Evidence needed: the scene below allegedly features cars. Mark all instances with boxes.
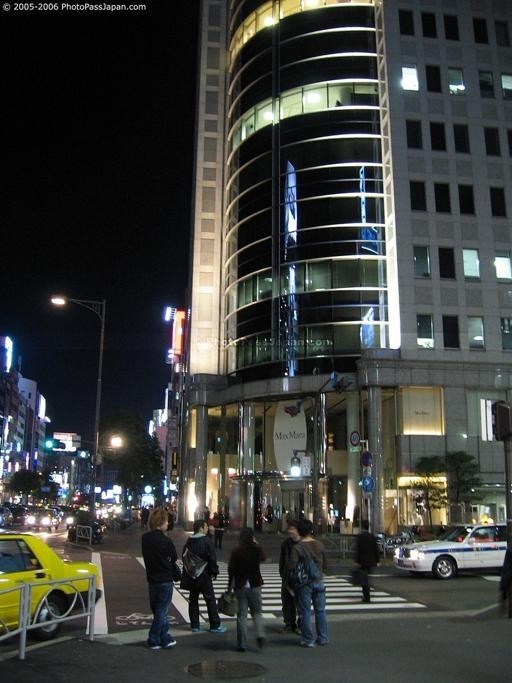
[0,534,102,635]
[393,522,508,578]
[0,502,124,532]
[370,531,406,553]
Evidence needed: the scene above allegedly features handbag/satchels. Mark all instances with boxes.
[207,517,220,527]
[146,546,322,617]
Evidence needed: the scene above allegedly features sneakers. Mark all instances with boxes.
[149,624,330,652]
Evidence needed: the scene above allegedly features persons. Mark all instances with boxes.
[214,511,225,548]
[142,510,180,650]
[228,527,266,652]
[132,505,174,532]
[256,504,287,536]
[182,519,227,633]
[436,526,467,541]
[194,507,201,522]
[288,519,329,647]
[202,507,211,538]
[413,526,425,536]
[311,520,321,537]
[278,520,303,635]
[355,520,378,603]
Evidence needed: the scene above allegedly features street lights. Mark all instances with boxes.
[291,449,319,535]
[51,295,106,540]
